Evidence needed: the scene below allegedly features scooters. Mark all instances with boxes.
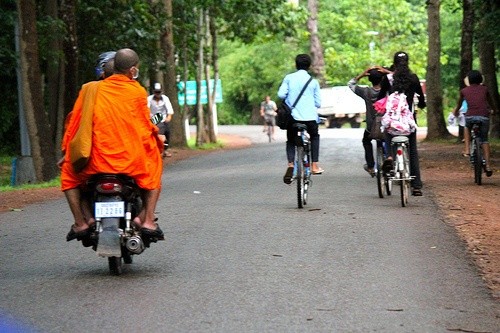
[88,113,170,277]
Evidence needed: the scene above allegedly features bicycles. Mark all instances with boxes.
[284,123,322,208]
[262,115,275,142]
[363,102,423,208]
[454,112,494,186]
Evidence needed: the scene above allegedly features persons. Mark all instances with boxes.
[452,71,496,176]
[148,84,174,158]
[56,47,164,242]
[351,48,427,197]
[276,53,324,184]
[261,96,277,140]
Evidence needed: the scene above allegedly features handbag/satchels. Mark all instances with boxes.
[70,115,94,171]
[274,102,294,130]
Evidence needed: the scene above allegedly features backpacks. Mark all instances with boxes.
[380,92,417,136]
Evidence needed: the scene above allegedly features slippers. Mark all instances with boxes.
[66,227,93,242]
[131,221,140,231]
[141,223,164,240]
[89,221,95,228]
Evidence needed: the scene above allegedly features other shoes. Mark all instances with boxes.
[486,170,493,177]
[459,153,471,159]
[364,164,376,177]
[411,188,423,196]
[283,166,294,184]
[312,167,324,175]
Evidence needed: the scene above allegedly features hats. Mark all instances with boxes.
[153,83,162,92]
